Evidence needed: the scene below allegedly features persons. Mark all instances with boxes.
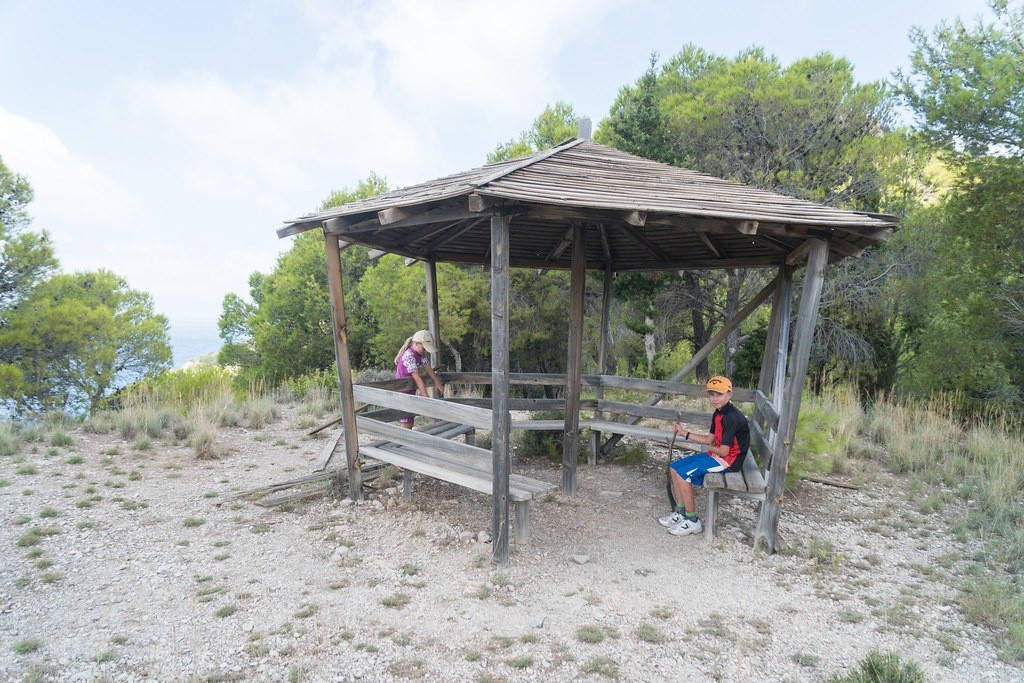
[659,376,751,536]
[394,330,445,430]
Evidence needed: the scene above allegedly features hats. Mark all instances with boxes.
[412,329,438,353]
[706,376,732,394]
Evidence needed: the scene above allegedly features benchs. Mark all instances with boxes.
[351,372,779,542]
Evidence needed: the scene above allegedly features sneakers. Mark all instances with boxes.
[668,517,702,536]
[658,512,685,528]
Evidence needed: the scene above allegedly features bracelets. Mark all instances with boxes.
[685,432,690,440]
